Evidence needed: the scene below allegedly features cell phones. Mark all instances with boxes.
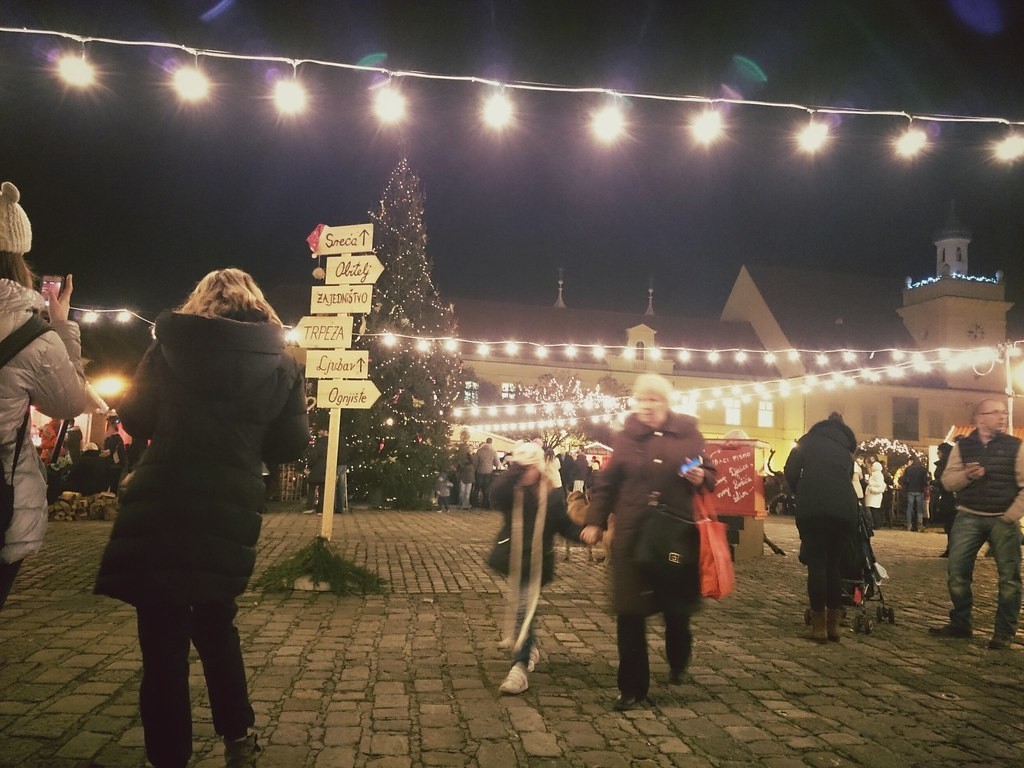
[679,455,704,476]
[37,273,66,320]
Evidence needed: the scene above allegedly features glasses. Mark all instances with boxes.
[982,409,1009,417]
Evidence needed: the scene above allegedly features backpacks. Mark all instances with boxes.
[0,311,56,550]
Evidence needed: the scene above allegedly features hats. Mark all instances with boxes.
[938,443,952,452]
[513,442,545,466]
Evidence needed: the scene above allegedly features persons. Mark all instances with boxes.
[0,182,85,622]
[765,398,1024,650]
[580,370,722,708]
[36,270,348,768]
[452,427,601,693]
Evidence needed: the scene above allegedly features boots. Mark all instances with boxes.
[826,607,840,641]
[812,607,828,643]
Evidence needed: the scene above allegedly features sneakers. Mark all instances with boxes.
[499,665,529,694]
[527,646,539,672]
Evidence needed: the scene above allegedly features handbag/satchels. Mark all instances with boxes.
[923,500,930,518]
[692,481,735,600]
[869,479,887,493]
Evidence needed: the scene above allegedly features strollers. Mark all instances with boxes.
[803,497,895,634]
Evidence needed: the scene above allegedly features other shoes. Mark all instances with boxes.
[989,635,1012,648]
[223,732,261,768]
[614,687,649,709]
[927,623,973,637]
[669,650,692,682]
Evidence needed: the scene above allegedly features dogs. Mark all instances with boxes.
[564,489,616,567]
